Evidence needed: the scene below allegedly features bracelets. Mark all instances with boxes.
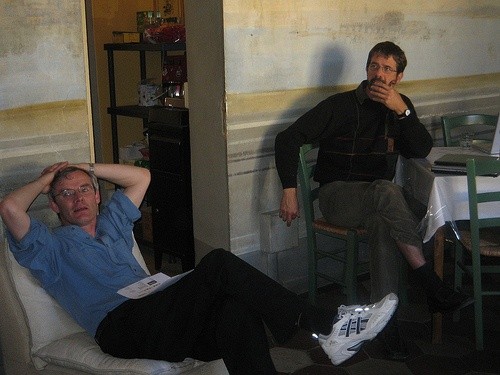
[88,163,95,174]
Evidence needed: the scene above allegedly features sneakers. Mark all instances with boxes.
[316,292,400,365]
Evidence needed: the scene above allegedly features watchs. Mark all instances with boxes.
[397,109,411,118]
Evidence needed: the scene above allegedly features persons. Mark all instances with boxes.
[275,40,477,360]
[0,161,399,375]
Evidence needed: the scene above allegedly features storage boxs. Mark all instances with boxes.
[111,32,141,43]
[164,97,185,107]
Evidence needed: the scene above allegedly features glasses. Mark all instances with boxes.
[54,185,97,201]
[369,62,397,75]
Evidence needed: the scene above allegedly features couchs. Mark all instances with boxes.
[0,203,228,375]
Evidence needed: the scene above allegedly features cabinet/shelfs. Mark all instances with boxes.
[102,42,193,272]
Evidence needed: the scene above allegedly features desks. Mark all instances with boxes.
[394,145,500,345]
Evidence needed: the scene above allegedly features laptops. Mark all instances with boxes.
[431,154,500,177]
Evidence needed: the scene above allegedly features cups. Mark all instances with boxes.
[459,132,474,150]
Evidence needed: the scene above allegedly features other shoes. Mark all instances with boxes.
[380,315,410,359]
[405,271,476,312]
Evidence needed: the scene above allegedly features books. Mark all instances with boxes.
[112,31,141,44]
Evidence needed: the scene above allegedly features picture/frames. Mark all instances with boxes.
[153,0,183,17]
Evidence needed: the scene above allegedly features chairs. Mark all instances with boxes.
[295,142,371,307]
[441,112,499,147]
[440,154,500,351]
